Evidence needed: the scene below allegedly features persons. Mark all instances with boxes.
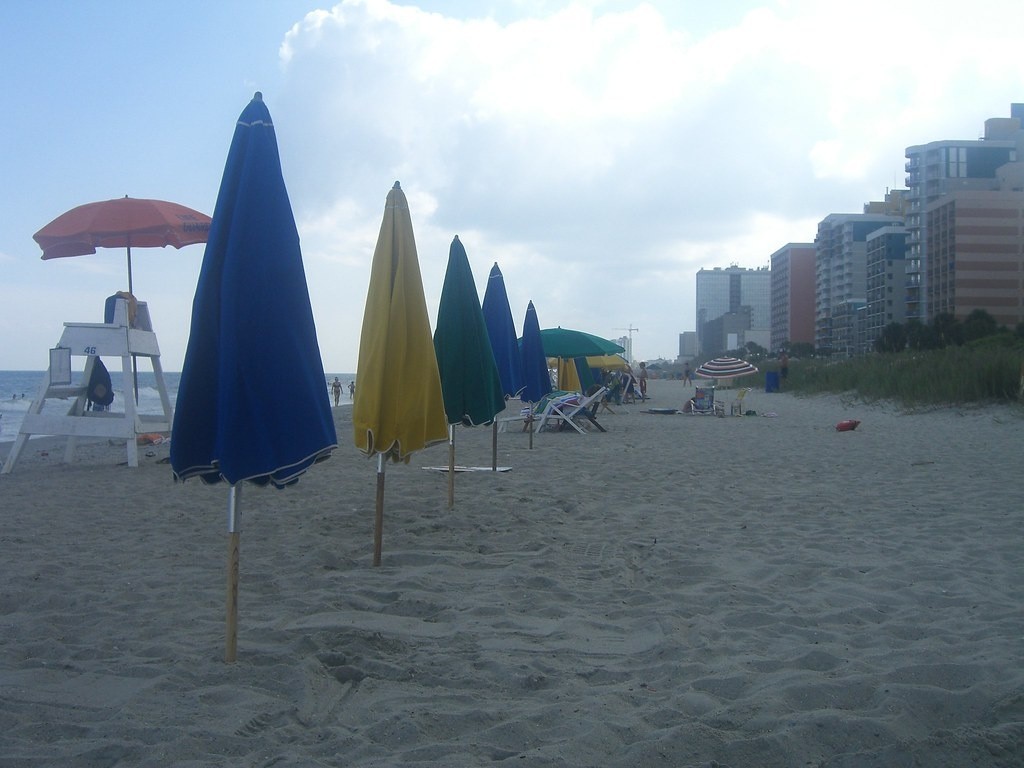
[778,348,789,393]
[639,362,648,402]
[619,372,636,404]
[331,377,343,407]
[348,381,355,399]
[682,397,705,413]
[605,375,622,406]
[683,363,692,387]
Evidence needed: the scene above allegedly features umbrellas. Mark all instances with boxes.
[695,355,759,402]
[482,262,552,471]
[353,181,450,567]
[433,235,506,506]
[170,92,338,662]
[32,194,212,407]
[517,326,638,424]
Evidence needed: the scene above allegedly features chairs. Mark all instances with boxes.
[498,371,638,435]
[691,386,716,416]
[104,294,125,324]
